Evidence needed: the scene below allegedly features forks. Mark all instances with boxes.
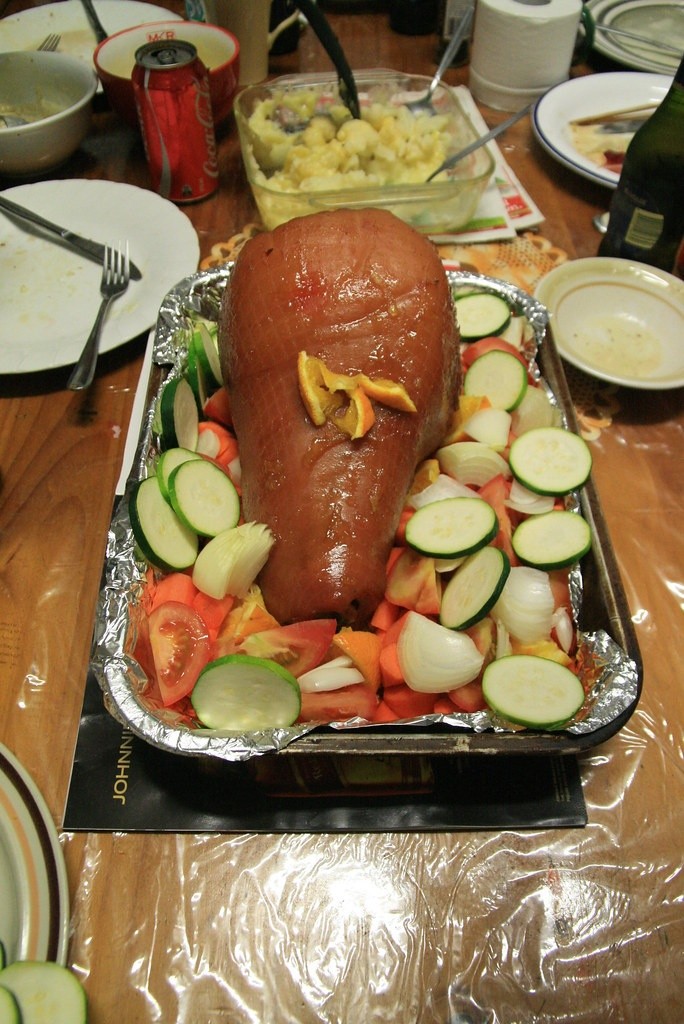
[65,238,131,392]
[36,34,61,52]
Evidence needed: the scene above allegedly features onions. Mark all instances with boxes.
[192,315,571,693]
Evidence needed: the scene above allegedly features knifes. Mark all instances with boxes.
[0,195,145,281]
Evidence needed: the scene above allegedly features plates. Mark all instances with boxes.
[578,0,684,75]
[91,273,647,765]
[0,0,185,94]
[534,255,684,390]
[528,72,675,187]
[0,179,202,375]
[0,739,71,973]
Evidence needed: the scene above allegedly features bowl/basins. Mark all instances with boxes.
[233,68,497,236]
[93,22,240,125]
[1,50,98,178]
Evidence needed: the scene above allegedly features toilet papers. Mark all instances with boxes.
[467,0,584,112]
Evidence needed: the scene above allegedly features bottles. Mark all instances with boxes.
[597,56,684,275]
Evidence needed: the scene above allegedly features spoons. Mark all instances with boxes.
[397,6,475,119]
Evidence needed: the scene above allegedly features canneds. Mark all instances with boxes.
[131,39,219,205]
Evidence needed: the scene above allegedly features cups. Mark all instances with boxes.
[185,0,271,85]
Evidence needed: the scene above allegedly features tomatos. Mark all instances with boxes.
[462,337,540,389]
[148,383,572,729]
